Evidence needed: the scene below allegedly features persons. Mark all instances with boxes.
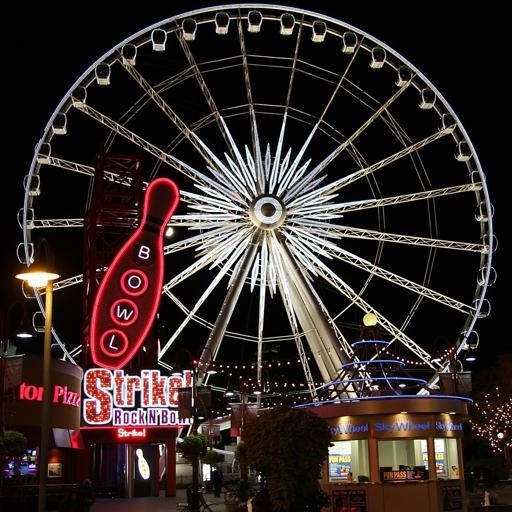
[212,466,224,498]
[79,474,96,512]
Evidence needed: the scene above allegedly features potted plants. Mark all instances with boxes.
[175,435,208,512]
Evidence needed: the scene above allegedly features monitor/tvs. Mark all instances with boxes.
[413,465,425,474]
[379,467,392,480]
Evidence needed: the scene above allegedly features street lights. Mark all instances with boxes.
[170,346,218,489]
[225,371,263,491]
[14,264,61,507]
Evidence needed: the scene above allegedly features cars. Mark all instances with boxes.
[465,461,500,490]
[0,460,38,475]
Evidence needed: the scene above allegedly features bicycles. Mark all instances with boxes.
[224,487,257,502]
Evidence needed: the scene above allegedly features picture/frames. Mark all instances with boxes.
[47,463,62,478]
